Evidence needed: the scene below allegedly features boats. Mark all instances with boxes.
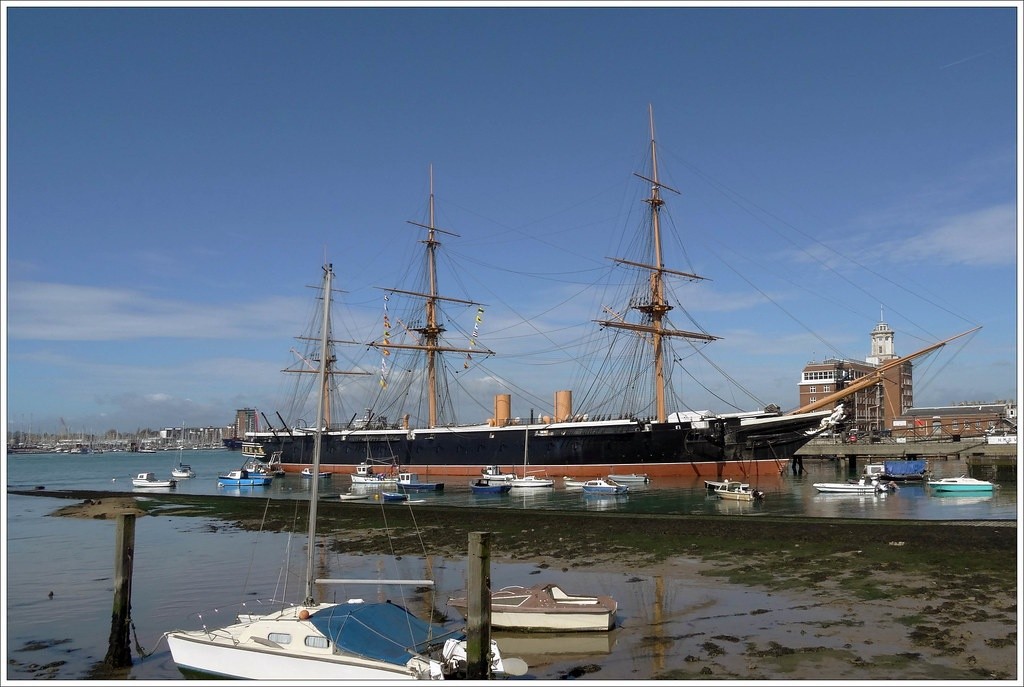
[446,585,617,631]
[132,455,993,502]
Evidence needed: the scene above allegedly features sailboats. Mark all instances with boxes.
[164,238,502,679]
[244,104,985,486]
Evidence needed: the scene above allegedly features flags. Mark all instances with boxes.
[464,307,484,370]
[379,294,392,389]
